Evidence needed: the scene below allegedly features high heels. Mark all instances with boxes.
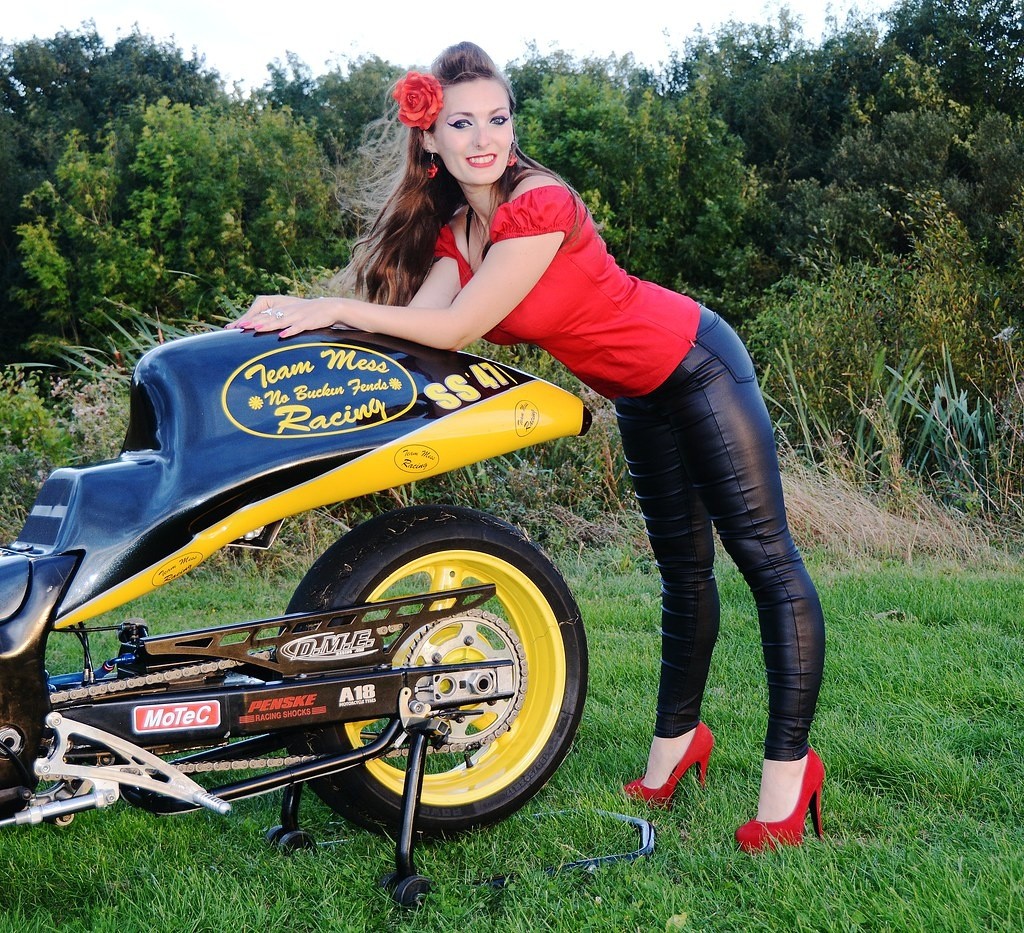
[623,720,714,811]
[736,745,826,856]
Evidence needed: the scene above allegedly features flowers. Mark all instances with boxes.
[393,71,444,131]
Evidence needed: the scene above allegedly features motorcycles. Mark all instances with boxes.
[0,325,661,911]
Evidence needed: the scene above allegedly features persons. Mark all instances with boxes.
[224,43,824,853]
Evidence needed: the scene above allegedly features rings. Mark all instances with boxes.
[275,311,284,319]
[260,308,272,315]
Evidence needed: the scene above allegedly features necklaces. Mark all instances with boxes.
[473,211,484,249]
